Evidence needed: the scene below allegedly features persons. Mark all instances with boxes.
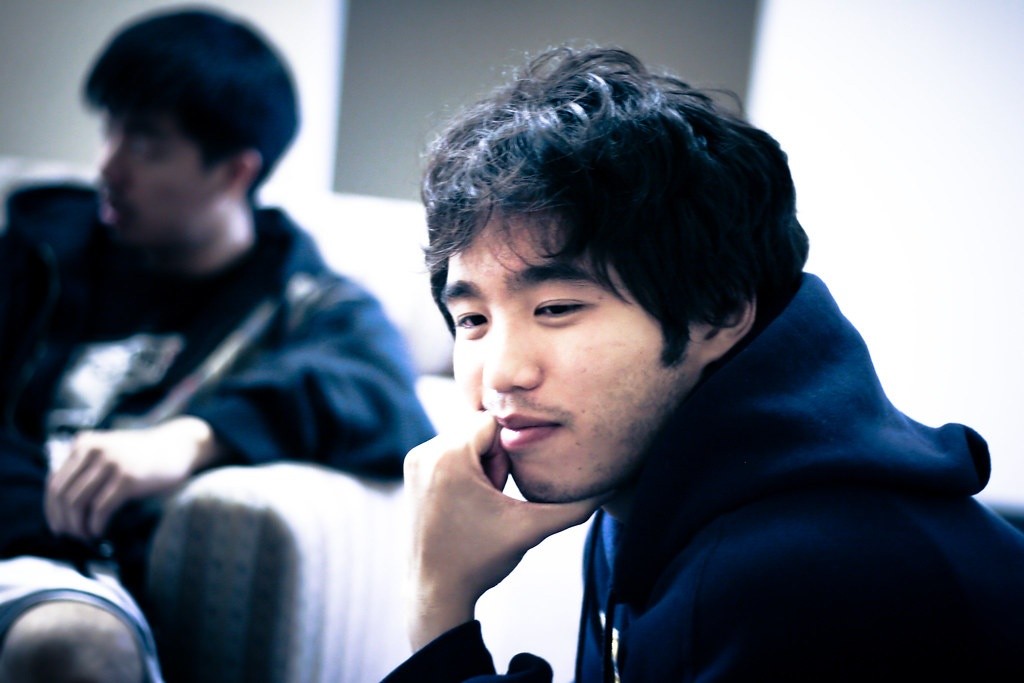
[375,43,1024,683]
[0,9,436,683]
